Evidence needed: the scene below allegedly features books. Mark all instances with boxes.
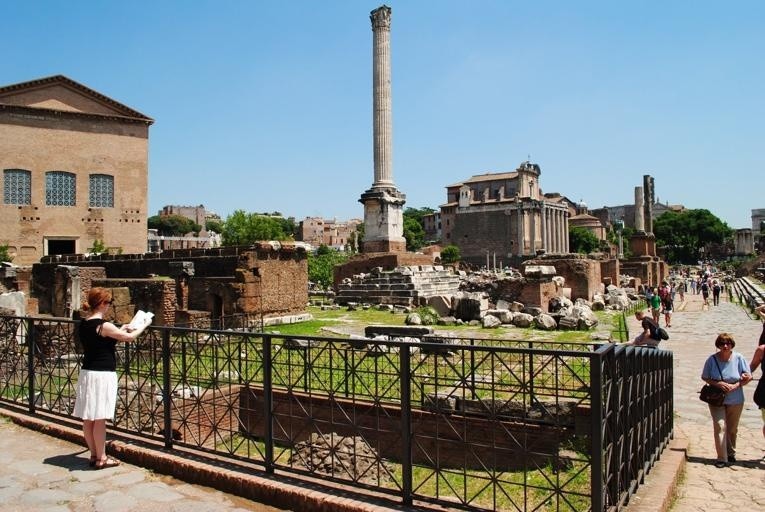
[125,309,155,331]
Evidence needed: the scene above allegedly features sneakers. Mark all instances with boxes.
[716,455,737,468]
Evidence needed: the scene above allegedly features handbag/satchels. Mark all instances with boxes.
[699,384,727,407]
[657,328,669,340]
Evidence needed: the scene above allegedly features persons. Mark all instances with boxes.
[645,264,727,328]
[71,287,153,469]
[702,334,753,469]
[606,309,663,352]
[739,327,765,435]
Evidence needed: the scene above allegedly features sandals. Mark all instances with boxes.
[89,455,121,470]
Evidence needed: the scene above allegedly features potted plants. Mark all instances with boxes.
[0,240,17,278]
